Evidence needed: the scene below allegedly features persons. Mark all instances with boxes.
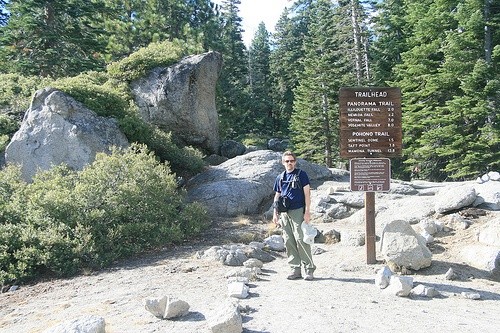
[273,151,316,280]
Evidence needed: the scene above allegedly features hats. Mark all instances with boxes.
[301,221,317,245]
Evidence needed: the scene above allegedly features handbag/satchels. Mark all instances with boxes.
[273,195,291,212]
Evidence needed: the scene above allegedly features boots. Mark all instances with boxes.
[286,267,302,280]
[304,269,314,280]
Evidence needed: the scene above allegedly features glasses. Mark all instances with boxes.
[284,160,295,163]
